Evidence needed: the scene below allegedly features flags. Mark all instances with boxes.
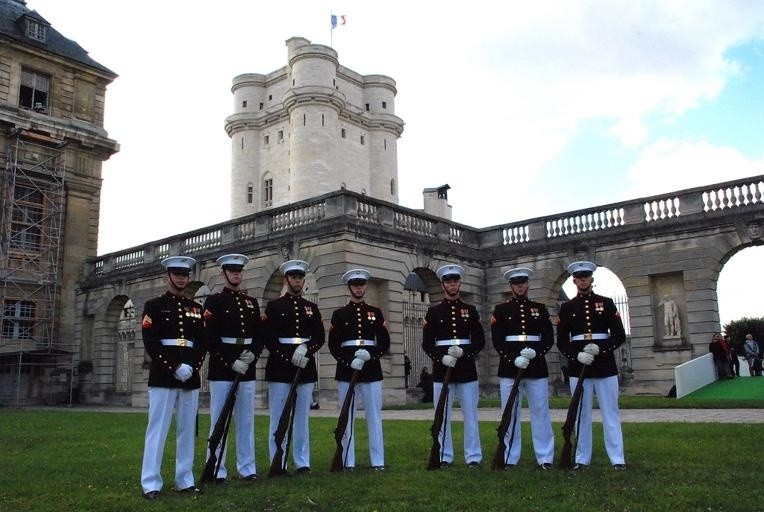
[331,15,346,28]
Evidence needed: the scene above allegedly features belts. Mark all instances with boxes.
[342,339,376,347]
[160,338,194,349]
[571,333,610,342]
[504,334,541,342]
[434,338,473,347]
[279,337,310,344]
[221,336,253,346]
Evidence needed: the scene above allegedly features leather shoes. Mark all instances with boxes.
[374,466,384,472]
[148,491,161,499]
[440,462,449,469]
[614,465,625,472]
[182,487,203,496]
[541,463,553,472]
[246,474,260,481]
[572,463,587,472]
[468,463,481,472]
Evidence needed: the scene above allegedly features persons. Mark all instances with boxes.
[709,333,740,379]
[405,350,412,389]
[328,269,389,471]
[422,264,486,470]
[556,261,627,472]
[490,268,555,472]
[203,254,264,483]
[743,334,763,376]
[657,294,677,336]
[141,256,207,500]
[419,367,433,403]
[261,260,325,474]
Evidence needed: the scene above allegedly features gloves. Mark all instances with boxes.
[520,347,536,359]
[172,363,193,383]
[354,348,371,361]
[350,358,364,371]
[583,344,600,356]
[240,349,256,364]
[448,346,464,358]
[576,351,595,366]
[290,345,310,369]
[442,355,458,368]
[514,357,530,369]
[231,360,249,375]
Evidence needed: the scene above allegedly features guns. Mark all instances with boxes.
[268,368,302,479]
[560,365,587,471]
[428,369,452,471]
[331,371,358,472]
[200,373,243,486]
[491,368,524,471]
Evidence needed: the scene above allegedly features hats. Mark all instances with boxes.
[568,261,597,277]
[436,265,466,283]
[504,268,534,284]
[216,253,249,271]
[160,256,196,272]
[341,269,371,285]
[279,260,310,276]
